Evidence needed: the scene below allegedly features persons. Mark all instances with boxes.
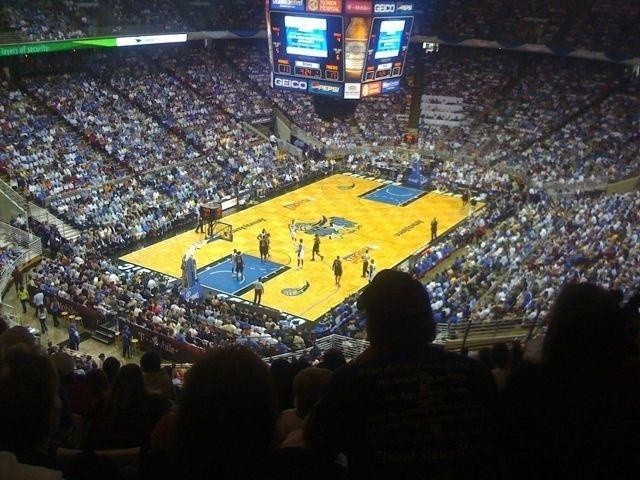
[1,1,640,477]
